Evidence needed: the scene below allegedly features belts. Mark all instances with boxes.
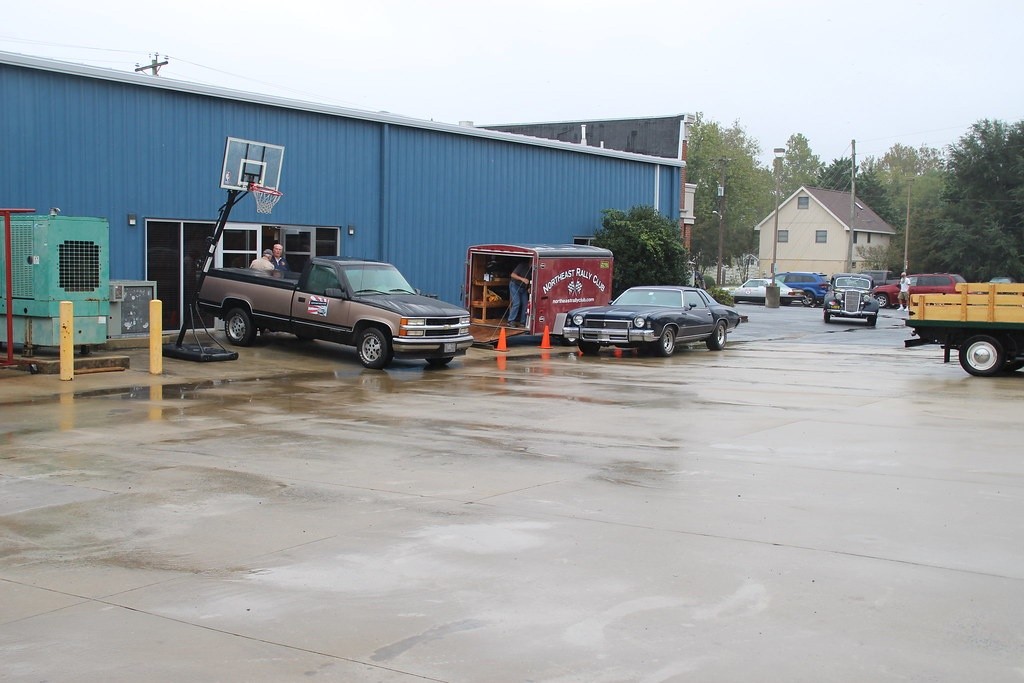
[512,281,525,288]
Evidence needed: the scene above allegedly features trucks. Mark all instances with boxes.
[904,283,1024,377]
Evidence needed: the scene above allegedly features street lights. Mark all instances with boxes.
[903,178,915,275]
[772,148,785,285]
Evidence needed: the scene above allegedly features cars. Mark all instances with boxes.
[988,275,1017,295]
[561,285,742,357]
[724,279,806,306]
[823,273,881,326]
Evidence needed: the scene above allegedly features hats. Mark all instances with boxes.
[263,249,273,256]
[901,272,906,276]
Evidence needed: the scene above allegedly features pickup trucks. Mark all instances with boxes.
[858,270,901,287]
[197,256,474,369]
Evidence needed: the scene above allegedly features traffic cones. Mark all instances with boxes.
[538,325,554,349]
[495,327,511,352]
[496,354,506,381]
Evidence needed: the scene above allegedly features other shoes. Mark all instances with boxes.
[897,307,903,310]
[520,323,525,327]
[904,308,909,311]
[507,321,516,328]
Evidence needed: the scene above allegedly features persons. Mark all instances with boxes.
[507,258,533,327]
[897,273,910,311]
[250,244,288,336]
[861,280,872,289]
[839,279,844,286]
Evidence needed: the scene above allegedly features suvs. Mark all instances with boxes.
[873,273,967,308]
[769,272,832,307]
